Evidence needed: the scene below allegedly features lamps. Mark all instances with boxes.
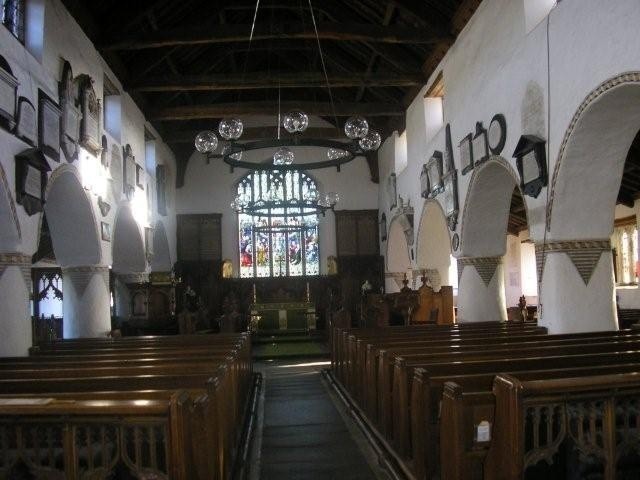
[231,190,339,216]
[195,1,381,172]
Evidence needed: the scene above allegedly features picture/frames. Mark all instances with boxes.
[460,134,472,174]
[451,233,459,252]
[39,89,62,162]
[101,222,110,241]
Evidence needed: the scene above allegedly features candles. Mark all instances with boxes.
[253,283,256,294]
[307,283,309,296]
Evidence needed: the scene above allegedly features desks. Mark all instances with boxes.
[262,302,305,329]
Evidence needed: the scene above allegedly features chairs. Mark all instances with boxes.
[331,309,640,480]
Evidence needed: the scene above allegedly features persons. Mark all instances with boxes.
[107,327,121,338]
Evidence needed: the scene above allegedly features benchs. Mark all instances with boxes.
[0,332,252,480]
